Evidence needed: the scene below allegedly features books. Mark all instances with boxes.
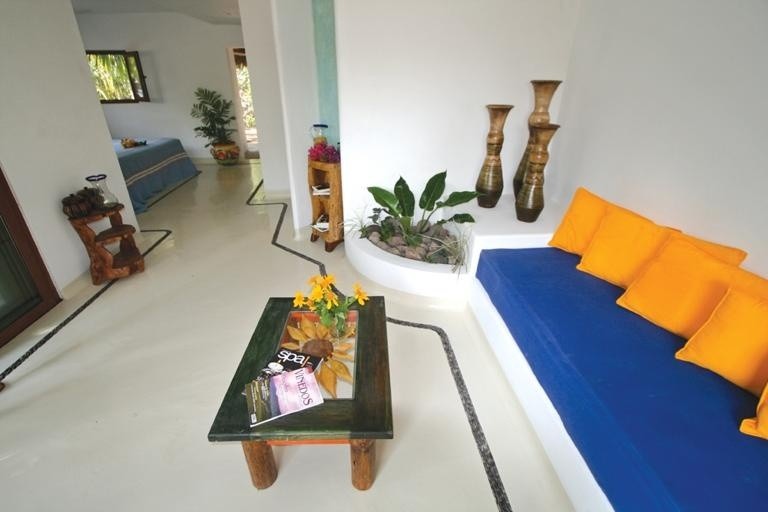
[244,366,325,429]
[241,345,324,399]
[309,182,330,235]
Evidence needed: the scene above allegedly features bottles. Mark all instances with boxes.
[84,173,119,211]
[308,123,328,148]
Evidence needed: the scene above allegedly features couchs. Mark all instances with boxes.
[470,243,768,512]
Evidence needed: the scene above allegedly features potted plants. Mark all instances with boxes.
[344,171,485,299]
[190,87,240,166]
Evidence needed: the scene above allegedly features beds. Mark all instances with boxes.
[113,138,198,215]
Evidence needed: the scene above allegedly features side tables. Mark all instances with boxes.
[445,185,564,312]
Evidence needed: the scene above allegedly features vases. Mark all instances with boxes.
[329,318,345,339]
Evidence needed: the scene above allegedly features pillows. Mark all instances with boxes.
[676,286,767,390]
[549,188,612,257]
[576,203,684,290]
[740,389,768,439]
[616,234,767,340]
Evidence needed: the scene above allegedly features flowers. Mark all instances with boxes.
[307,144,340,163]
[292,273,370,330]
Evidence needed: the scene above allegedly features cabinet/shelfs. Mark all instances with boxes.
[68,202,145,285]
[308,155,344,251]
